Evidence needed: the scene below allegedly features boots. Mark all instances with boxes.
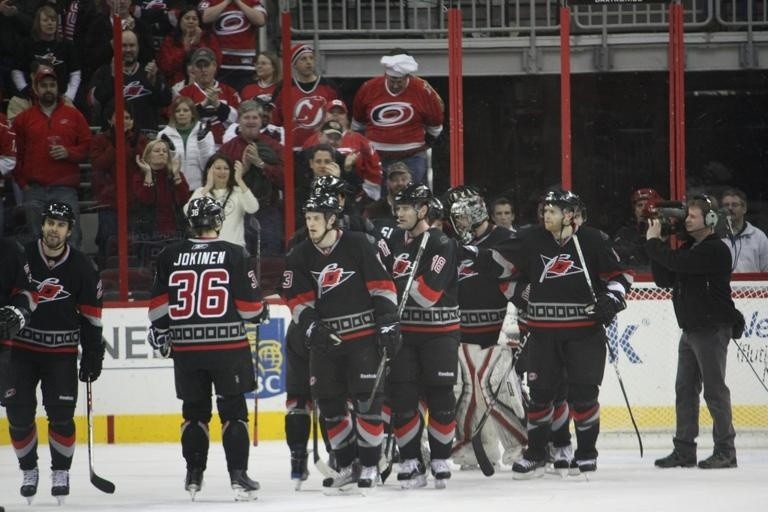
[699,451,737,468]
[655,437,697,467]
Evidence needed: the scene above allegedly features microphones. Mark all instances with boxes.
[687,223,714,232]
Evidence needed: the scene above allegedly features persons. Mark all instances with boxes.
[442,183,636,484]
[708,187,768,276]
[0,3,466,505]
[645,194,739,470]
[612,187,672,270]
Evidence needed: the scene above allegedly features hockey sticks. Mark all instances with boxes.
[86,376,117,493]
[312,409,332,476]
[249,215,262,446]
[572,233,644,465]
[472,330,532,475]
[356,232,431,414]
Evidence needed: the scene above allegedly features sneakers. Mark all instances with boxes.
[397,457,426,480]
[323,463,361,488]
[291,459,309,478]
[430,459,451,479]
[51,469,70,496]
[553,445,572,468]
[185,469,203,491]
[512,455,546,472]
[575,457,597,472]
[358,466,377,487]
[20,465,38,496]
[229,470,259,491]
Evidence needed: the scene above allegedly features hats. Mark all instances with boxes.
[255,93,276,110]
[322,120,343,136]
[324,98,348,113]
[387,161,408,176]
[291,44,312,66]
[191,46,216,65]
[35,68,58,82]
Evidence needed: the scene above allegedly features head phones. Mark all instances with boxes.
[694,193,718,229]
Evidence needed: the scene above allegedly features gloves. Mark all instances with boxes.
[303,319,345,351]
[79,343,105,382]
[0,303,32,340]
[594,290,626,327]
[377,321,404,364]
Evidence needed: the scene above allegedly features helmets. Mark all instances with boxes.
[41,200,76,227]
[302,193,339,212]
[444,184,481,216]
[631,188,661,202]
[573,205,589,226]
[543,190,580,207]
[311,175,345,193]
[449,195,489,232]
[392,181,432,204]
[184,196,225,229]
[428,196,444,211]
